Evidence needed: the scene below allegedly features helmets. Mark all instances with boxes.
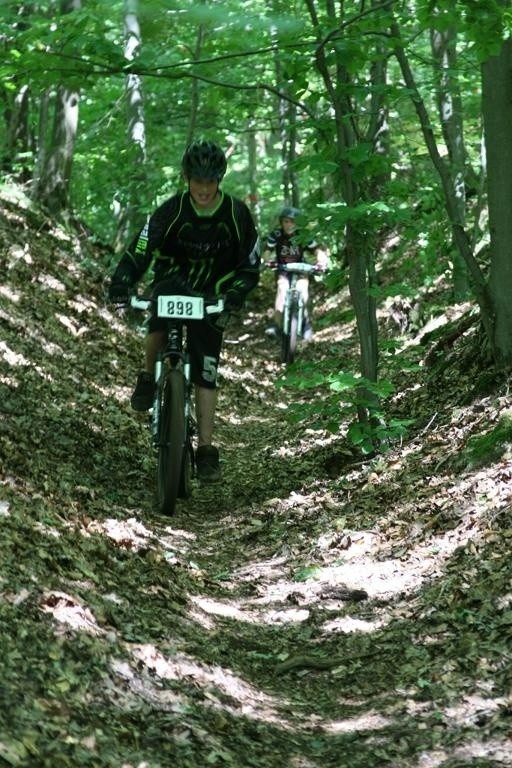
[180,138,227,180]
[280,207,300,220]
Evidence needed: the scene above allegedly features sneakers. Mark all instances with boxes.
[303,322,314,338]
[264,323,280,337]
[194,444,221,481]
[131,372,155,410]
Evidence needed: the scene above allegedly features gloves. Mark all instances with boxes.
[223,288,243,312]
[313,263,327,273]
[109,280,129,303]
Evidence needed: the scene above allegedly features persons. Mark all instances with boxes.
[265,206,330,343]
[108,139,265,486]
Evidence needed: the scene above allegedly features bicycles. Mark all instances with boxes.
[97,276,249,515]
[265,261,327,361]
